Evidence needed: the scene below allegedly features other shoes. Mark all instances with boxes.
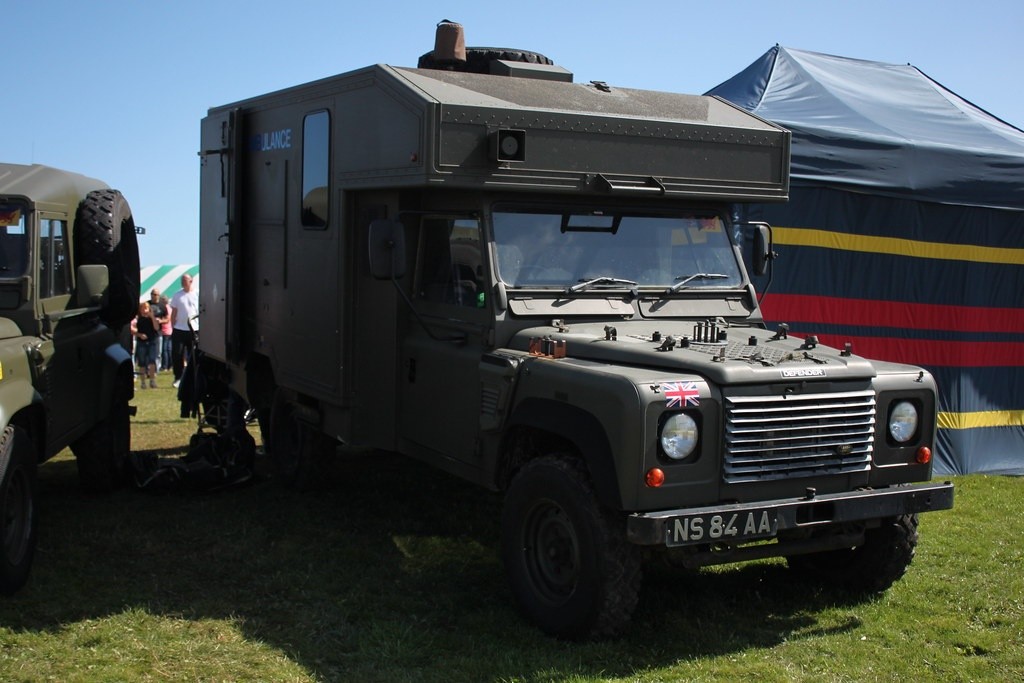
[141,384,146,389]
[150,381,157,387]
[172,379,181,388]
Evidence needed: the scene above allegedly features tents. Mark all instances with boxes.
[140,264,199,302]
[701,41,1024,478]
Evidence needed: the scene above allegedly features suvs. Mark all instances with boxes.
[0,162,146,598]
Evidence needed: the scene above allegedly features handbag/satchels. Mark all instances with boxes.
[189,425,223,452]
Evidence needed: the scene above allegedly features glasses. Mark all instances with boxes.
[151,294,158,296]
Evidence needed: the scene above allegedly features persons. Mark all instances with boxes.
[146,290,173,378]
[170,273,199,388]
[131,302,159,389]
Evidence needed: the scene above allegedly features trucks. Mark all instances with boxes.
[197,20,955,640]
